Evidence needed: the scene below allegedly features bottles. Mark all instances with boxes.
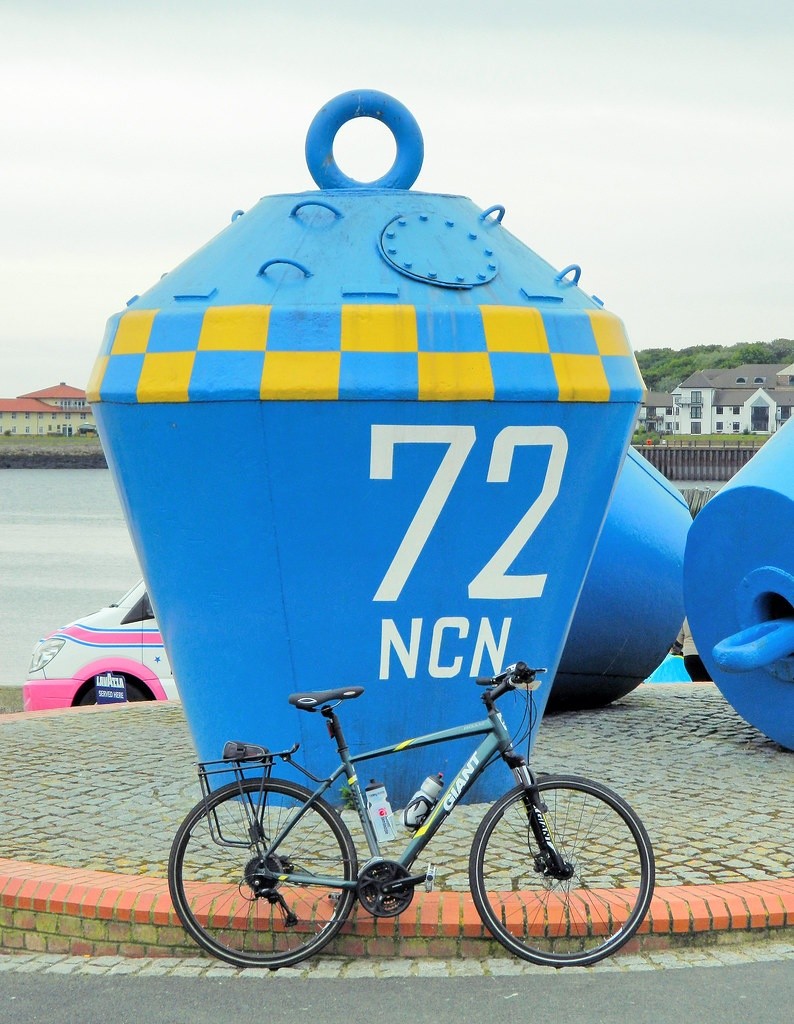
[398,772,445,833]
[365,778,399,844]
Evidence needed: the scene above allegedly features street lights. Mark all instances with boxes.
[672,404,679,441]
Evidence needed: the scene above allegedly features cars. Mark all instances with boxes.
[21,575,181,711]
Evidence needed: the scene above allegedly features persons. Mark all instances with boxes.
[672,616,713,682]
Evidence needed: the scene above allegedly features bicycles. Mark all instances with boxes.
[167,657,655,971]
